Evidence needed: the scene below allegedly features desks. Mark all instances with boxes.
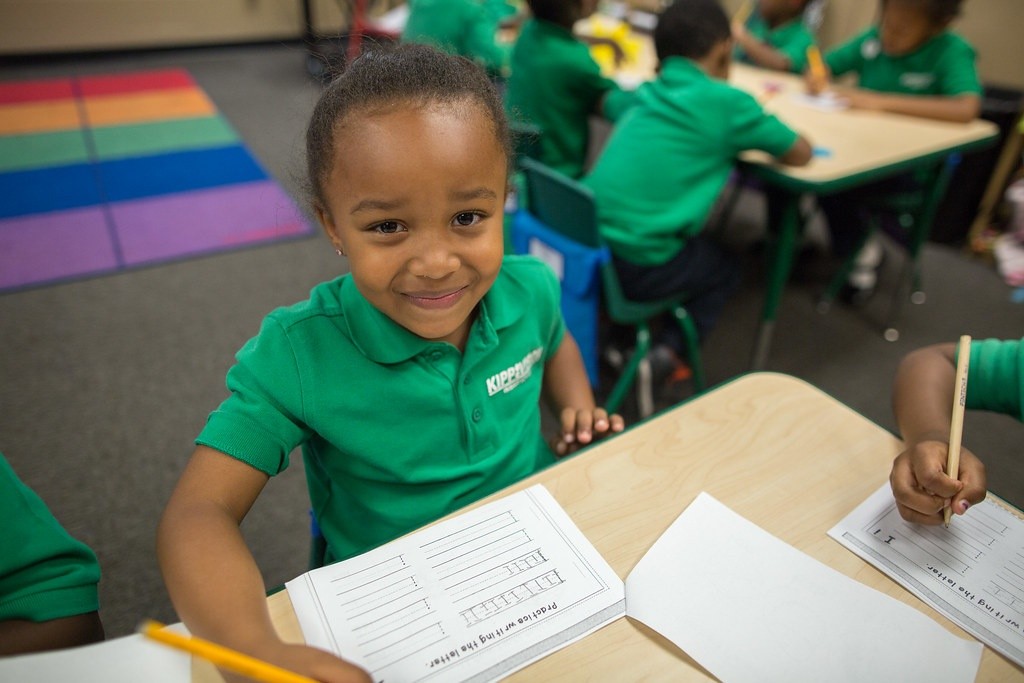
[192,371,1024,683]
[613,37,1003,372]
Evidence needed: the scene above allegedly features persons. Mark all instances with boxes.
[501,0,620,180]
[398,0,518,101]
[802,0,985,310]
[159,42,624,683]
[889,335,1024,524]
[728,0,819,278]
[581,0,813,389]
[0,451,102,658]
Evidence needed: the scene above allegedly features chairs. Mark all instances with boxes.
[506,114,715,425]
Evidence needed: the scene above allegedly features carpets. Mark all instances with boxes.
[0,68,319,296]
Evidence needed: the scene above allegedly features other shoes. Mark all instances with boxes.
[600,339,633,376]
[649,343,691,387]
[836,242,890,308]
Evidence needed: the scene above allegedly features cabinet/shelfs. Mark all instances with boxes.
[0,0,1024,90]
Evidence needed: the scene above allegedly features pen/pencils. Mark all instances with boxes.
[143,620,318,683]
[943,335,971,528]
[807,47,827,93]
